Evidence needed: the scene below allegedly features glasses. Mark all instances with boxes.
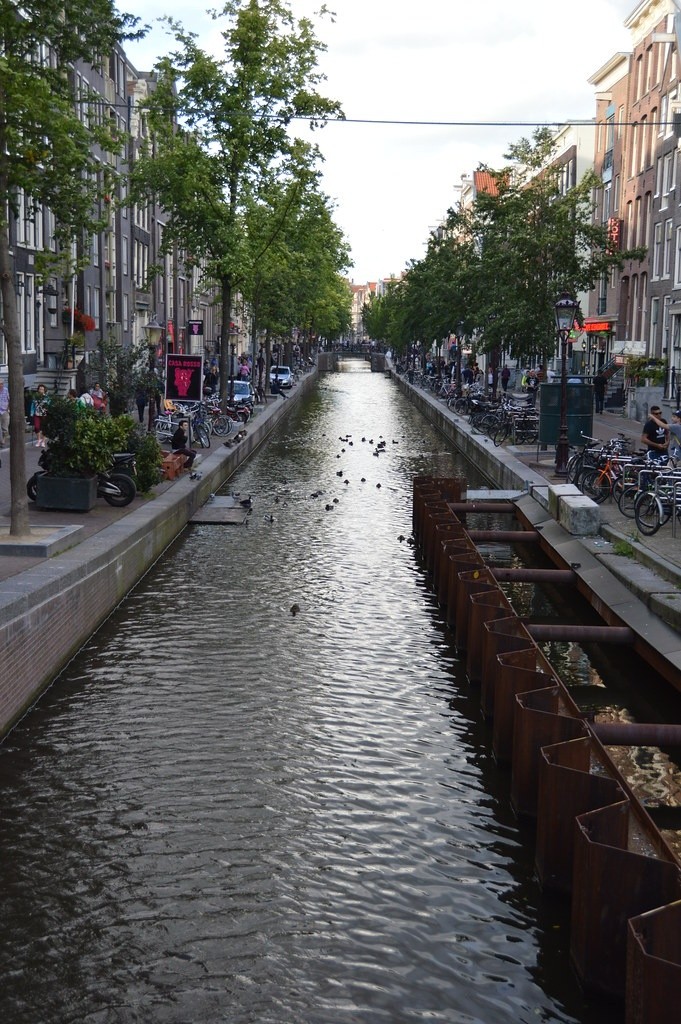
[651,412,662,415]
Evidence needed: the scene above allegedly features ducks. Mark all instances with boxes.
[290,604,300,616]
[189,430,448,547]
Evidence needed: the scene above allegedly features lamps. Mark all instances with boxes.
[582,338,587,354]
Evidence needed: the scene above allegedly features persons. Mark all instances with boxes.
[567,369,583,383]
[357,338,360,351]
[521,364,555,408]
[203,351,266,394]
[346,340,350,350]
[641,406,681,461]
[426,361,493,387]
[501,364,510,391]
[0,378,11,448]
[172,420,197,471]
[134,376,165,424]
[593,369,608,415]
[30,382,108,447]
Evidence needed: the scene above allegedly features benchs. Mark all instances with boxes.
[162,455,188,481]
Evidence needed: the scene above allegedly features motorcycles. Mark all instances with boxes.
[26,442,139,508]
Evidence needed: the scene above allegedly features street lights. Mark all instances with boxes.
[554,291,581,479]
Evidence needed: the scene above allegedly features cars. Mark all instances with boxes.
[154,395,254,449]
[270,366,295,388]
[227,380,256,402]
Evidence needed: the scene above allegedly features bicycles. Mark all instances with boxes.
[565,431,681,536]
[255,385,268,406]
[421,373,540,448]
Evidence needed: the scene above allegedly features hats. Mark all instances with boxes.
[672,410,681,418]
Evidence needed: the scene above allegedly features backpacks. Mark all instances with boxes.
[84,397,92,407]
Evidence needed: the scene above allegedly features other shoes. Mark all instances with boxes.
[184,466,197,472]
[1,441,6,448]
[596,410,603,414]
[35,442,46,448]
